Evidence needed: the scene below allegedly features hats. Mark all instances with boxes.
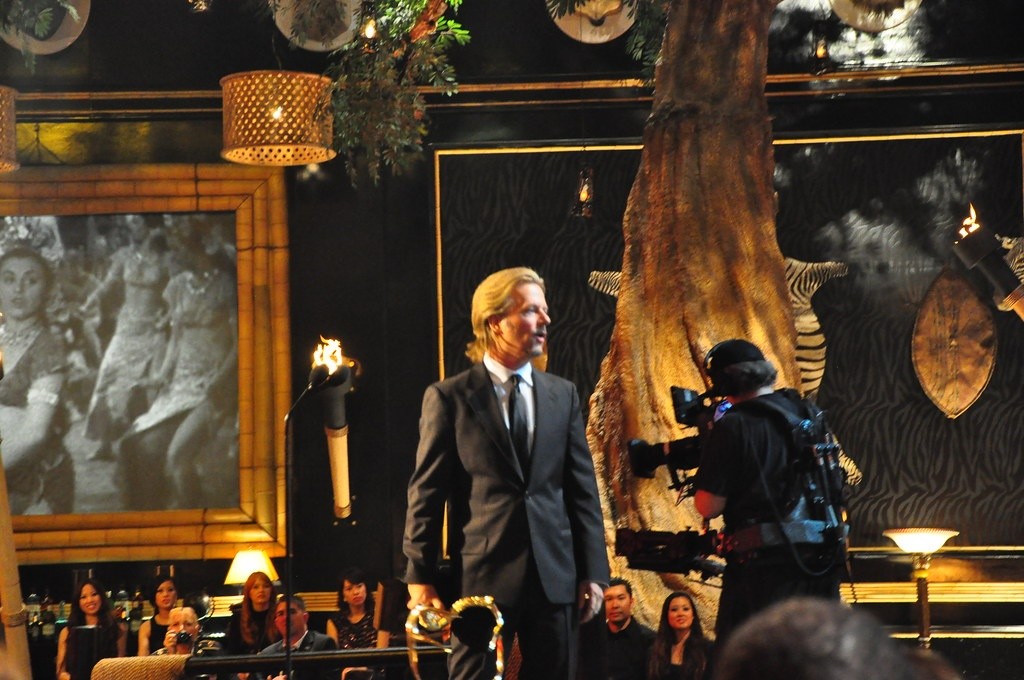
[711,339,766,370]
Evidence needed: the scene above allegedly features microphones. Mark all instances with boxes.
[306,363,330,388]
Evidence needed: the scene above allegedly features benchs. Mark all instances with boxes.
[840,573,1024,648]
[0,581,383,680]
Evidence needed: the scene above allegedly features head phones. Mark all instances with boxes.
[703,340,737,396]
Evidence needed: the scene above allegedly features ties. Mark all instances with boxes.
[509,374,529,470]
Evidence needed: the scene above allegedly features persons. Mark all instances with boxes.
[403,266,612,680]
[135,573,178,657]
[0,213,241,514]
[326,563,378,649]
[55,576,129,680]
[221,571,279,680]
[576,577,658,679]
[248,594,341,680]
[695,338,849,634]
[149,607,201,656]
[644,591,715,680]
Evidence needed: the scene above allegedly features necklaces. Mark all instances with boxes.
[0,321,46,347]
[186,268,220,295]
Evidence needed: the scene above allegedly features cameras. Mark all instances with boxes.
[174,631,191,643]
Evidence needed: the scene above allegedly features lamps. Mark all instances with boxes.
[881,527,960,652]
[216,0,339,168]
[224,552,281,595]
